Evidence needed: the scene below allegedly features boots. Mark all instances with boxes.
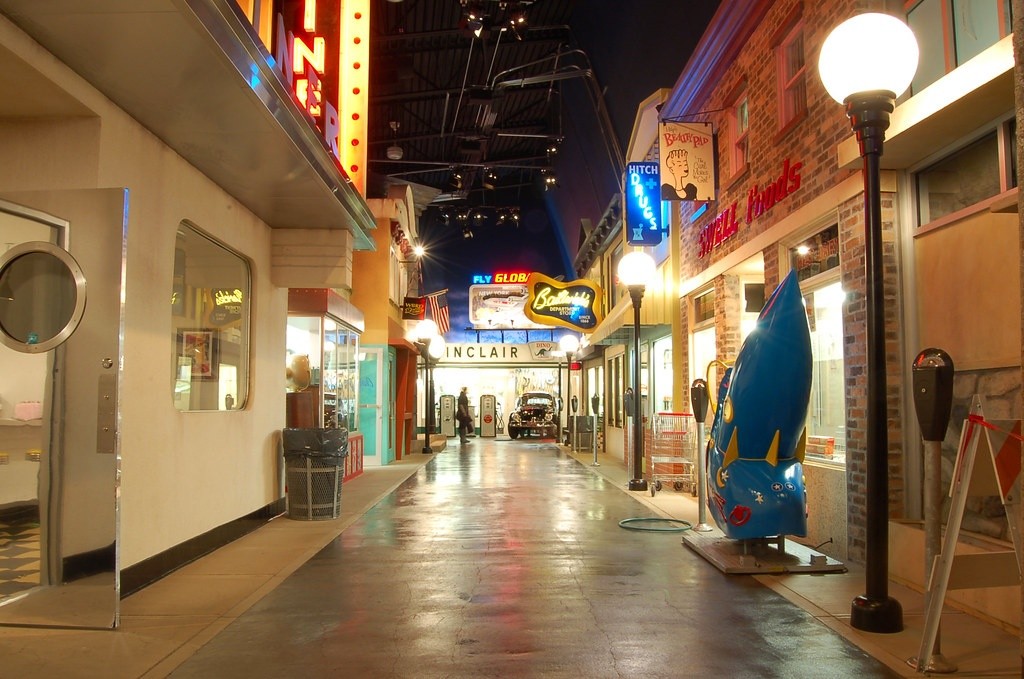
[460,433,470,443]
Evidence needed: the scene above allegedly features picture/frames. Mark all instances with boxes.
[175,328,220,382]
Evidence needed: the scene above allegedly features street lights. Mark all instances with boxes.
[816,11,921,634]
[416,317,439,455]
[558,335,580,446]
[427,334,446,433]
[617,250,657,491]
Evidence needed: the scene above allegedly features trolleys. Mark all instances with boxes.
[649,410,697,500]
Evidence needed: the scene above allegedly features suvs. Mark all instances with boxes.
[507,391,562,439]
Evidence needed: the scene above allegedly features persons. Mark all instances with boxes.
[456,386,474,445]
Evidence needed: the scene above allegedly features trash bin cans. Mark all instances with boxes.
[282,427,348,520]
[568,416,594,452]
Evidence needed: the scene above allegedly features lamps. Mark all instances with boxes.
[435,134,567,241]
[386,120,403,160]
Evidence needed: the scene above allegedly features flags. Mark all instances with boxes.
[428,291,451,335]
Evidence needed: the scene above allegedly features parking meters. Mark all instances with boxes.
[625,386,635,487]
[690,377,714,531]
[910,348,961,673]
[558,397,564,447]
[572,396,578,452]
[591,393,601,466]
